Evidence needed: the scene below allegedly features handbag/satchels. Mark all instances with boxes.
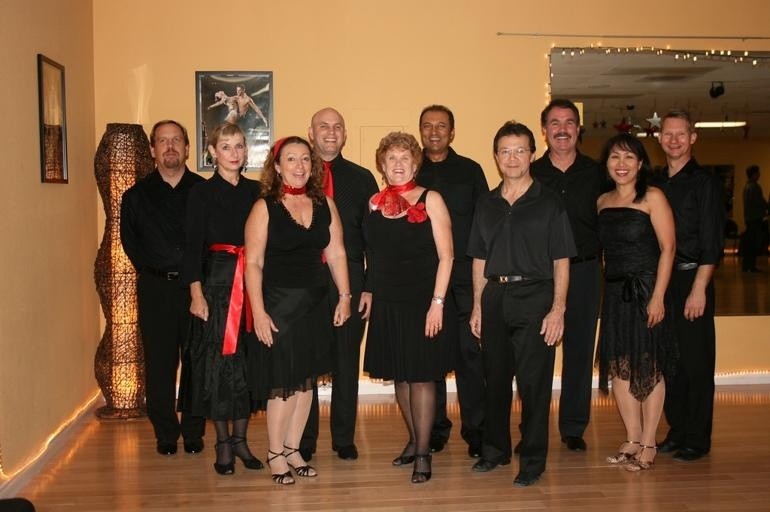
[430,294,447,303]
[338,290,351,301]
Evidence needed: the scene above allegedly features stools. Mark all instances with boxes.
[139,264,182,284]
[487,274,530,286]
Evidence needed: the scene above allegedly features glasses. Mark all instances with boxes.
[84,121,159,421]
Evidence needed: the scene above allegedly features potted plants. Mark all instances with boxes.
[194,70,274,173]
[38,53,68,184]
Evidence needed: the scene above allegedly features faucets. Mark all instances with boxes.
[607,441,658,472]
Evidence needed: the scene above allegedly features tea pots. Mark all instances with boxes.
[545,45,770,319]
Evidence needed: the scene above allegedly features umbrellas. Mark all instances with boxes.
[393,429,586,487]
[157,418,357,485]
[660,433,709,463]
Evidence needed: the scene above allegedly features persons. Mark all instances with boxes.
[516,99,595,455]
[358,131,457,484]
[649,110,727,462]
[468,119,575,488]
[119,120,208,455]
[206,90,241,129]
[410,102,489,456]
[223,85,271,125]
[242,135,354,484]
[183,120,266,476]
[300,107,380,462]
[741,159,767,275]
[595,136,678,481]
